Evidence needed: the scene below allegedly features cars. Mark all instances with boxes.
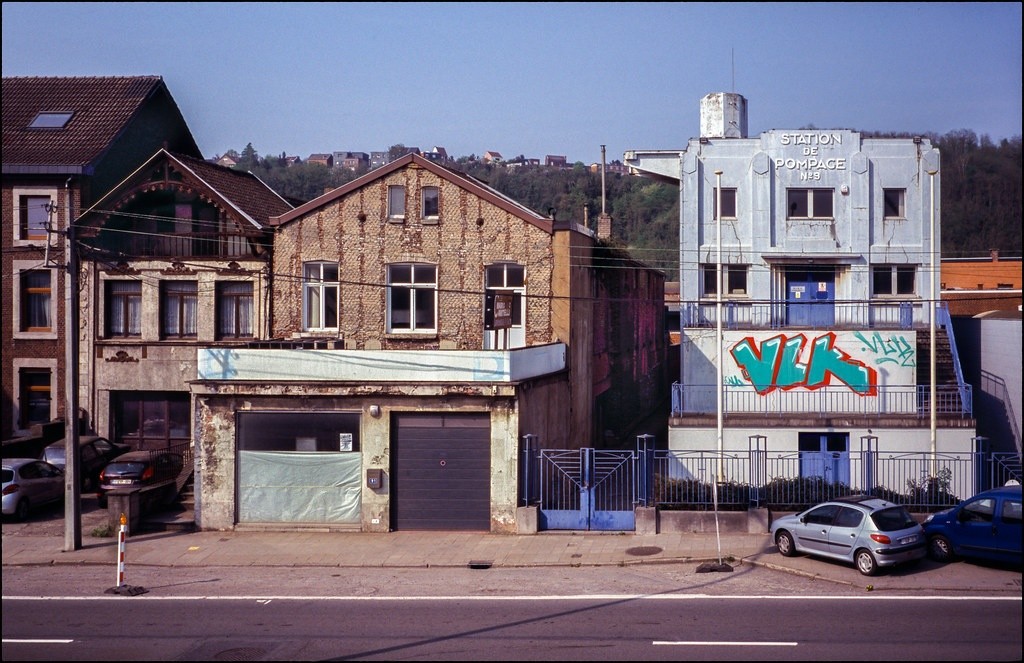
[0,458,66,520]
[39,435,131,493]
[97,451,182,509]
[921,485,1023,562]
[770,495,927,576]
